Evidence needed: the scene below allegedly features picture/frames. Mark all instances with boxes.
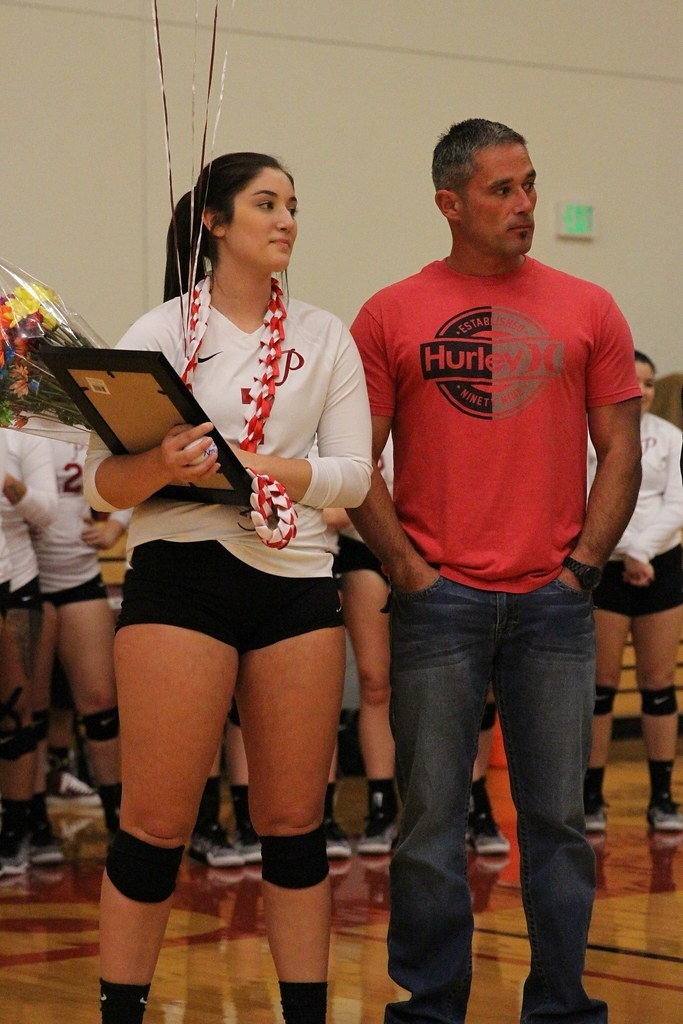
[41,346,253,504]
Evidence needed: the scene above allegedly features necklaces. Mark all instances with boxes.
[170,269,297,551]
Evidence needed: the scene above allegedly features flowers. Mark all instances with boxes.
[0,279,93,432]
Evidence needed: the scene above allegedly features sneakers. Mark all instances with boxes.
[465,809,510,856]
[329,862,350,886]
[46,767,102,810]
[327,817,353,859]
[1,829,29,874]
[189,824,246,867]
[646,800,682,830]
[587,833,605,846]
[2,878,26,888]
[209,867,262,884]
[362,857,390,884]
[233,827,262,864]
[475,857,509,874]
[583,794,607,831]
[649,834,678,854]
[33,828,66,865]
[35,869,65,886]
[358,814,405,853]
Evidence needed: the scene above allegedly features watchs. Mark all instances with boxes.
[564,557,602,590]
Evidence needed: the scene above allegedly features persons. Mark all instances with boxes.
[584,350,683,834]
[352,118,644,1024]
[0,406,133,876]
[191,425,511,866]
[81,152,373,1024]
